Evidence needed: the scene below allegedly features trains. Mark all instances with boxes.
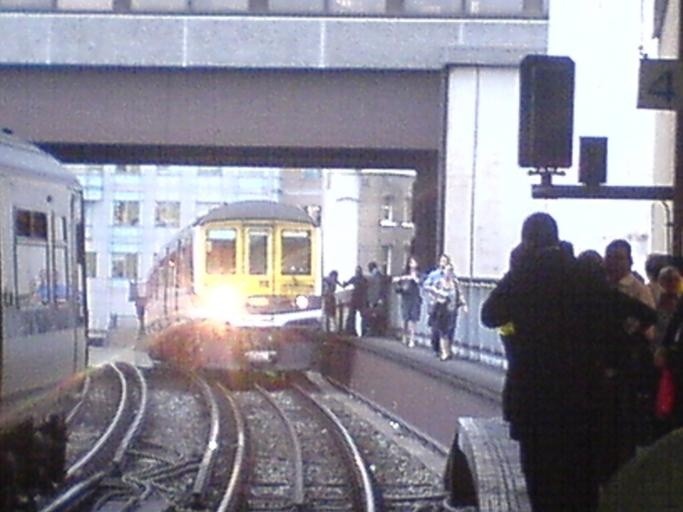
[142,199,323,380]
[0,128,89,511]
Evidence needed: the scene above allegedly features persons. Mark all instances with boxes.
[321,254,469,362]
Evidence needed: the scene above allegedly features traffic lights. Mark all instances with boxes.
[574,134,604,184]
